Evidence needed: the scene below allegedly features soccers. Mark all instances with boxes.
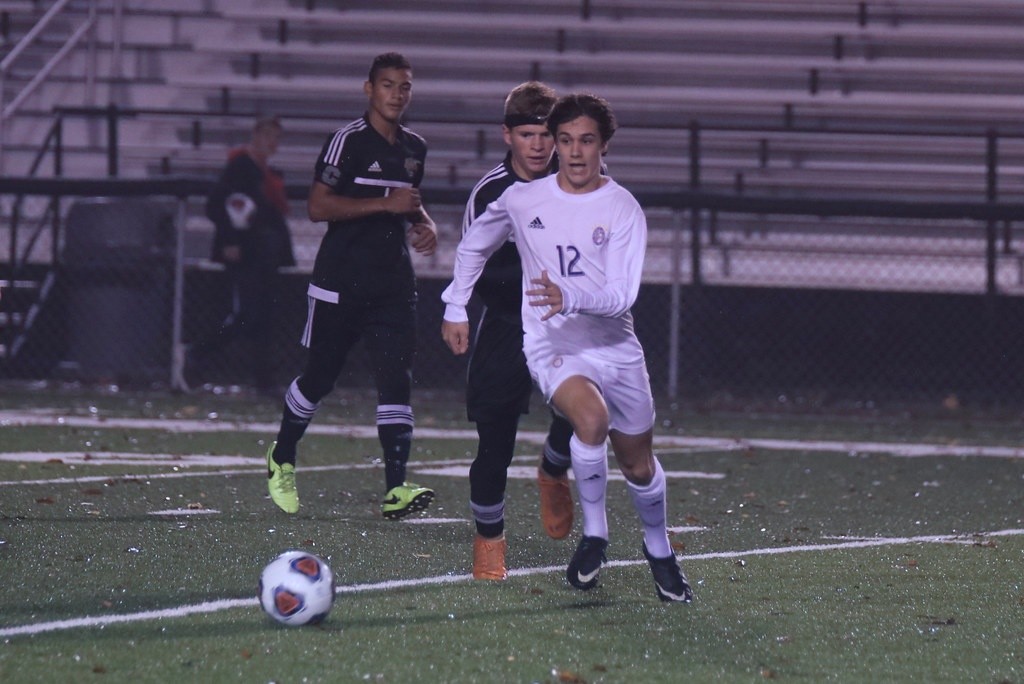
[256,549,336,627]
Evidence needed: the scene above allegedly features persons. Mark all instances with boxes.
[441,93,693,605]
[464,82,607,583]
[266,51,436,521]
[179,115,297,396]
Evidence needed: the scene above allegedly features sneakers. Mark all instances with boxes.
[267,440,299,514]
[382,483,435,520]
[566,533,609,590]
[471,532,508,582]
[536,452,572,537]
[642,536,693,605]
